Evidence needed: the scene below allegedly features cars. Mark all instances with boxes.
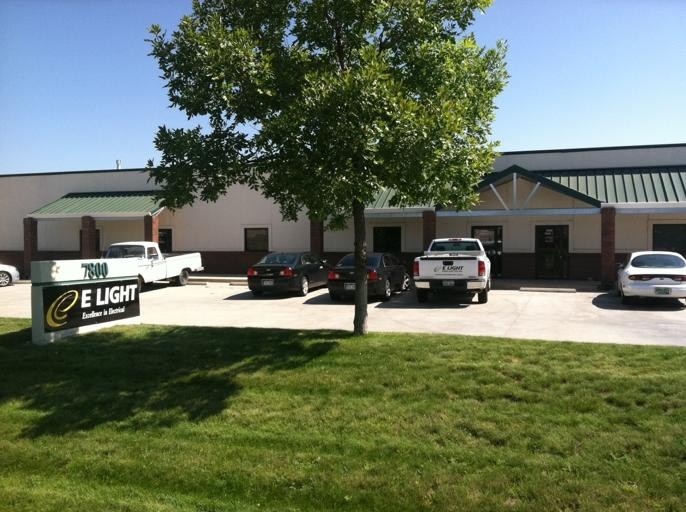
[0,262,20,287]
[246,250,329,298]
[615,249,686,304]
[327,254,413,300]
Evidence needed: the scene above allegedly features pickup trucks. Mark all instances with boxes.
[101,240,204,293]
[412,235,496,307]
